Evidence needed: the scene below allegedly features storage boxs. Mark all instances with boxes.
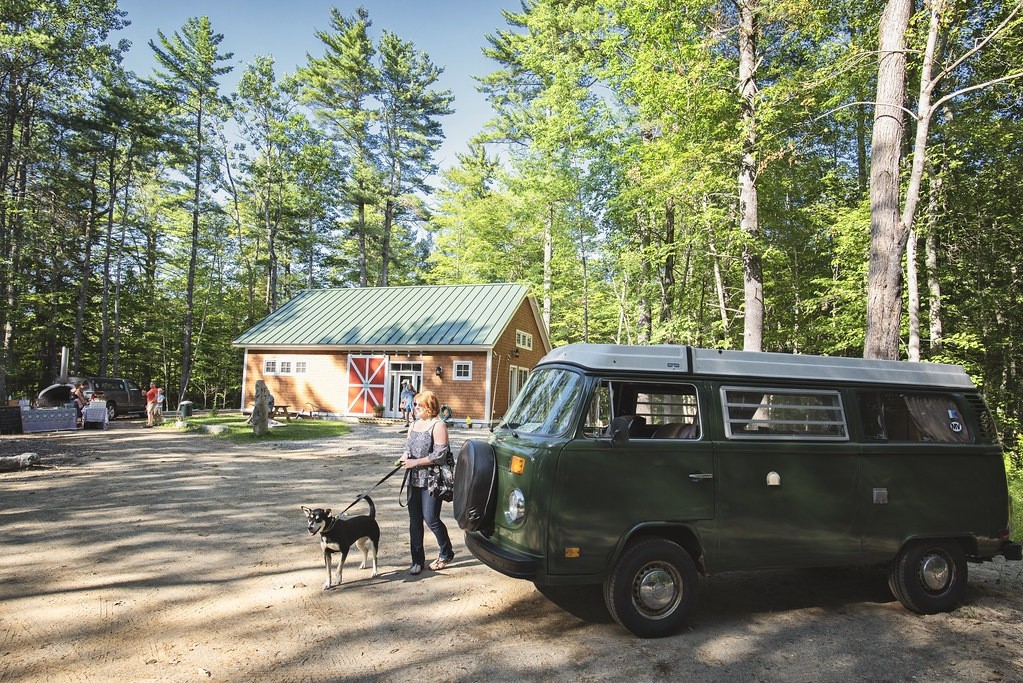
[9,399,30,411]
[89,401,107,408]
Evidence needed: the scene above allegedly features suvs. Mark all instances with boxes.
[52,376,148,422]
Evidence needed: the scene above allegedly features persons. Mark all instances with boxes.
[153,388,165,425]
[69,379,90,430]
[399,379,417,427]
[397,390,456,575]
[141,381,158,428]
[245,390,274,424]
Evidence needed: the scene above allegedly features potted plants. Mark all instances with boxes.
[372,405,385,418]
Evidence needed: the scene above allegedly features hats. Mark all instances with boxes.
[401,379,407,383]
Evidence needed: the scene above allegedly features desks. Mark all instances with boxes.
[271,406,292,423]
[21,409,78,433]
[82,408,109,431]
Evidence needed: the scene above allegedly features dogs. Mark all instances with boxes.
[300,493,381,591]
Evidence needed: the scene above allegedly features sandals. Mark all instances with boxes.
[428,551,454,571]
[409,562,424,575]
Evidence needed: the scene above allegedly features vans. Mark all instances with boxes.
[449,341,1022,638]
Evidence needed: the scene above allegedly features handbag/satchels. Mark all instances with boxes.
[427,421,456,502]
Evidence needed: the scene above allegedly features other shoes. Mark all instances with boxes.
[404,424,409,427]
[399,417,405,419]
[143,424,153,428]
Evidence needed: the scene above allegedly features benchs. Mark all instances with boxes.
[267,411,298,417]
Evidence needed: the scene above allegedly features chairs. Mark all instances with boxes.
[650,423,698,439]
[604,415,646,438]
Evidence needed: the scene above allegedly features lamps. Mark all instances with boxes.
[436,366,443,375]
[512,348,520,359]
